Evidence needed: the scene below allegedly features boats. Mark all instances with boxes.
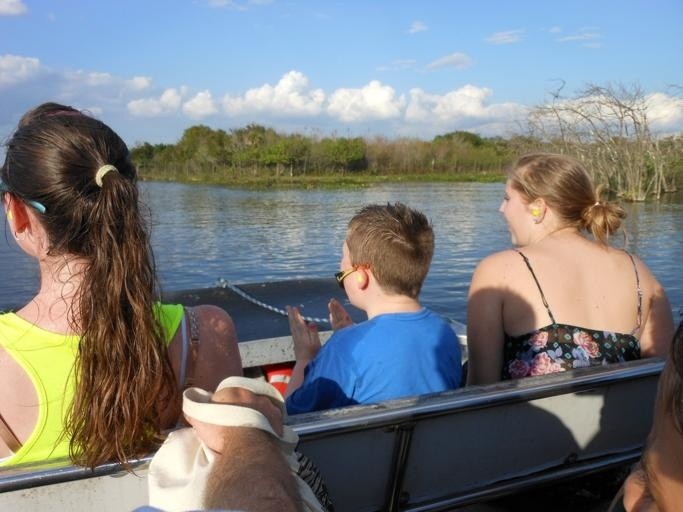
[0,273,668,510]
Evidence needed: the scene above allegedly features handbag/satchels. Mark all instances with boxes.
[147,375,335,512]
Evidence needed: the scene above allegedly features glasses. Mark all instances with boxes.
[335,267,357,288]
[1,170,49,214]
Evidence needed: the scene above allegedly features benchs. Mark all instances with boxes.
[1,356,666,512]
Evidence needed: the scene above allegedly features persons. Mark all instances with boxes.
[458,145,671,382]
[619,308,682,511]
[279,201,459,413]
[1,98,240,465]
[177,384,304,512]
